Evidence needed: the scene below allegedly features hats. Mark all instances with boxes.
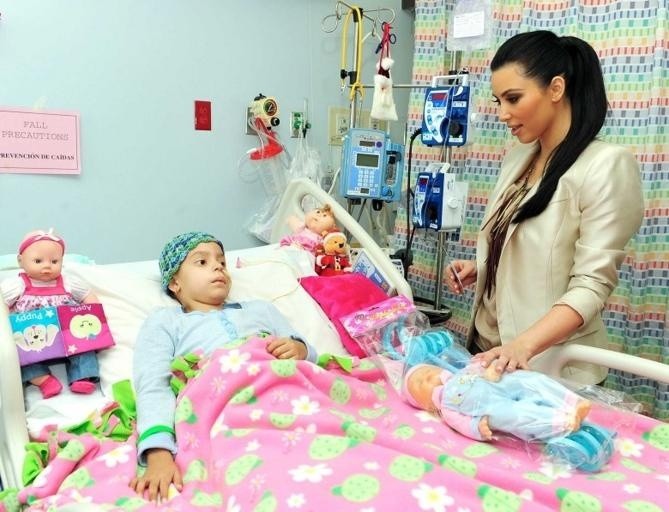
[157,230,223,295]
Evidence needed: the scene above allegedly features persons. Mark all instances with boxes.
[1,228,104,400]
[127,230,318,507]
[443,31,644,398]
[396,361,592,441]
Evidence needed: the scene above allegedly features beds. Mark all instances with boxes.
[0,174,668,512]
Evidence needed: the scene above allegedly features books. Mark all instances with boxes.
[354,250,397,298]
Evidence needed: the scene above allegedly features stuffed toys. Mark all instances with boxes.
[281,204,333,253]
[313,227,352,276]
